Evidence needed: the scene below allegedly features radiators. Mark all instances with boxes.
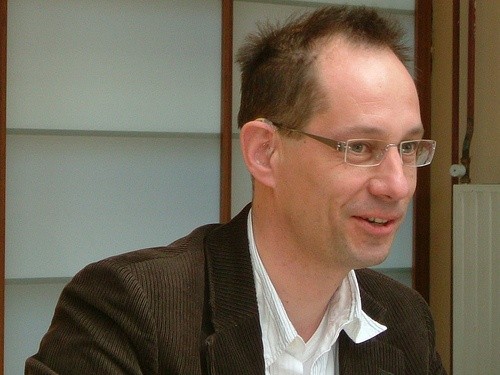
[451,182,500,375]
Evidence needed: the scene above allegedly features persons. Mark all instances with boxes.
[24,7,446,375]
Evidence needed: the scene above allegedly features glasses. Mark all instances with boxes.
[256,118,437,168]
